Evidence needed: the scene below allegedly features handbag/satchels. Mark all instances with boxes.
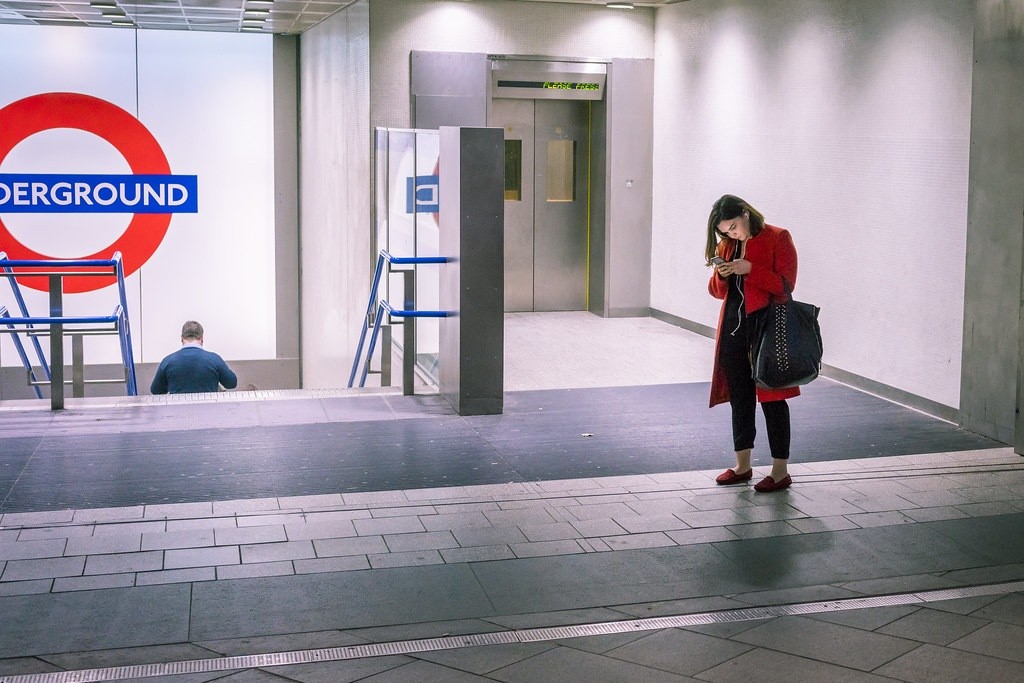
[747,276,822,390]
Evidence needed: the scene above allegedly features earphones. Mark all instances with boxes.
[744,213,749,219]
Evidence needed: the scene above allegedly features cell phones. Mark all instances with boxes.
[711,256,726,265]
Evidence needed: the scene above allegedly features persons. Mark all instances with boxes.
[704,194,798,492]
[149,320,238,395]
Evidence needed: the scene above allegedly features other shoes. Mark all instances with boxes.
[716,468,752,484]
[754,473,792,492]
